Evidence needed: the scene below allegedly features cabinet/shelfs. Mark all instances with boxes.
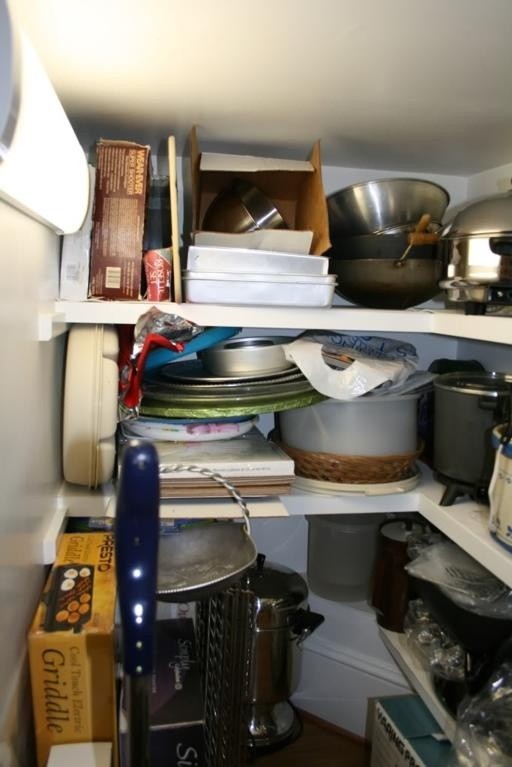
[39,297,511,767]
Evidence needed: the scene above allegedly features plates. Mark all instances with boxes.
[123,414,260,442]
[138,359,313,401]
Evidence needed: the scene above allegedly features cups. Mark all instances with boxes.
[377,516,428,633]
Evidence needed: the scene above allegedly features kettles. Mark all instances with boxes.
[193,551,326,749]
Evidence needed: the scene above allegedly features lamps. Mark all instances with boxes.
[0,0,91,238]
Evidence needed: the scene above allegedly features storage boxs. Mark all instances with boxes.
[183,125,335,258]
[26,531,124,767]
[84,136,154,303]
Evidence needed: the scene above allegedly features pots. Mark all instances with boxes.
[430,368,511,484]
[328,233,449,260]
[440,178,512,288]
[328,212,449,308]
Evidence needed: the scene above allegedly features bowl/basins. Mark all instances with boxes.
[197,335,297,377]
[326,177,451,232]
[277,390,424,454]
[202,178,290,234]
